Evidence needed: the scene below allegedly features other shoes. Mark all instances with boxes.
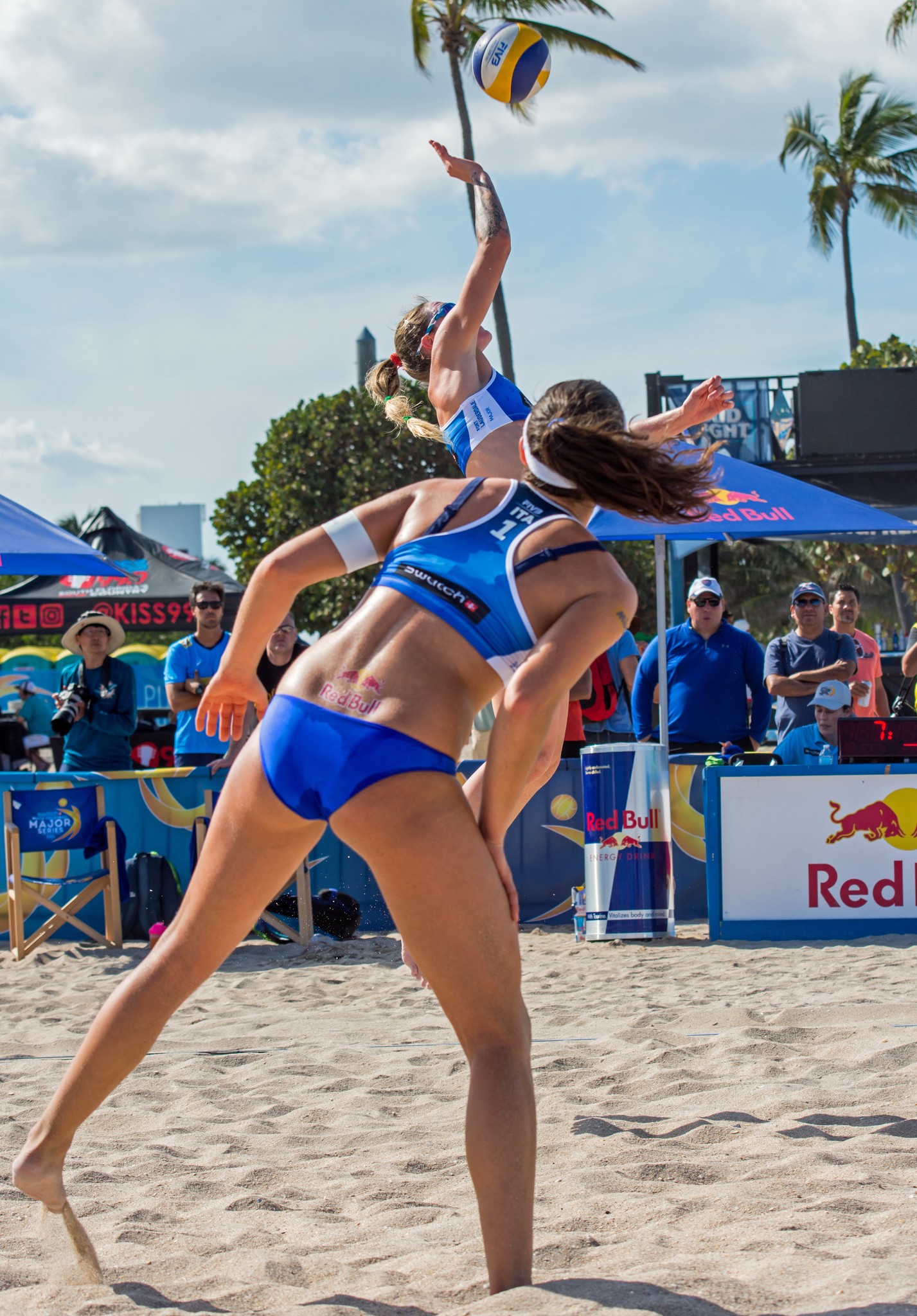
[36,763,51,772]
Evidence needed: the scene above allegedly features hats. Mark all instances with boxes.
[16,680,37,693]
[807,679,851,711]
[733,620,750,632]
[688,577,724,599]
[61,610,126,657]
[722,609,734,620]
[792,582,826,605]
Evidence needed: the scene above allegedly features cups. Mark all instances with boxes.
[149,922,167,950]
[857,680,872,707]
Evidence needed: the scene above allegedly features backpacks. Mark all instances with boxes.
[579,648,626,724]
[255,887,360,946]
[120,852,184,944]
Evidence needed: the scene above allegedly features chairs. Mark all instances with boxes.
[190,790,314,946]
[4,785,122,960]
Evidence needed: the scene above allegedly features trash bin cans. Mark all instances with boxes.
[579,742,675,941]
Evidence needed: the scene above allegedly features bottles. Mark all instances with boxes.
[819,745,833,765]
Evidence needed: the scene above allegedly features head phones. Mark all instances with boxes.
[22,681,29,696]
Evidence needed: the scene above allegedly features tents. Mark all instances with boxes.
[0,507,246,633]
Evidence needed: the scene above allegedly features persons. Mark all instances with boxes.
[11,379,730,1297]
[256,612,312,703]
[51,610,138,773]
[901,622,917,718]
[364,140,734,988]
[584,632,642,745]
[0,679,177,772]
[561,668,592,759]
[762,581,858,746]
[723,609,758,714]
[769,679,856,766]
[828,583,891,764]
[163,584,232,768]
[630,577,772,755]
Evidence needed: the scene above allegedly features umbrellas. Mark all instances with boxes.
[587,440,917,750]
[0,494,129,576]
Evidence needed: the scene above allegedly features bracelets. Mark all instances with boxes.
[322,514,381,576]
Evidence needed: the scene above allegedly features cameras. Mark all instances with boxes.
[51,682,92,736]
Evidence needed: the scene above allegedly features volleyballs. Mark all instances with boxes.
[472,22,551,104]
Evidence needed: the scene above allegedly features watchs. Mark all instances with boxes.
[194,684,205,699]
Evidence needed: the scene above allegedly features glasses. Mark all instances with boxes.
[194,600,223,610]
[274,624,296,633]
[794,598,825,608]
[853,638,865,659]
[690,596,721,607]
[416,302,457,357]
